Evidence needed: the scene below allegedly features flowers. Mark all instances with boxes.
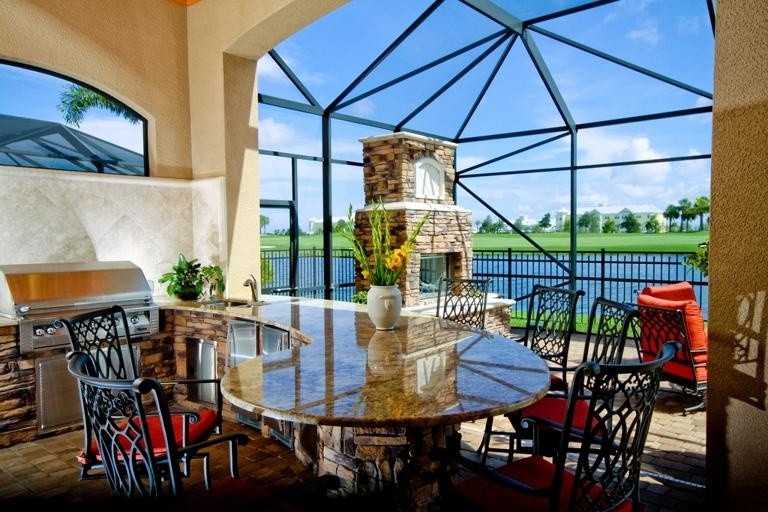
[332,194,432,286]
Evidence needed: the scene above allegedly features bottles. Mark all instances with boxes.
[367,330,404,377]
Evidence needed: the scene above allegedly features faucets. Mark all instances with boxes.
[242,273,259,302]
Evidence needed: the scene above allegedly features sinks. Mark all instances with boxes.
[214,298,271,309]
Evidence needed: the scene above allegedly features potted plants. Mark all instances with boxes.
[157,253,225,301]
